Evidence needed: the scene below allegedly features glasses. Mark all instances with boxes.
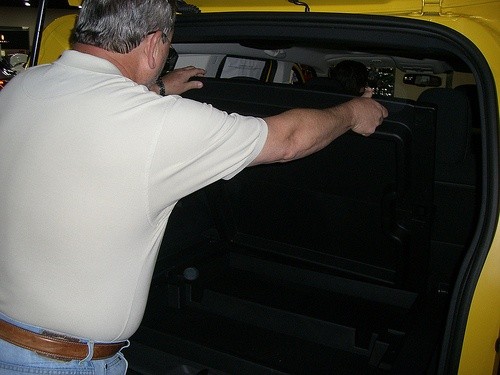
[145,28,177,61]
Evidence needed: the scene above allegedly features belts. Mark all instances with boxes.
[0,317,128,362]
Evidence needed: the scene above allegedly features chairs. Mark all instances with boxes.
[413,87,473,185]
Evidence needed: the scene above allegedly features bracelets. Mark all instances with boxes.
[156,78,165,97]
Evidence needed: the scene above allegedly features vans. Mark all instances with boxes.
[25,0,500,375]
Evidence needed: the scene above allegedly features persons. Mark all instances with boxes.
[0,0,388,375]
